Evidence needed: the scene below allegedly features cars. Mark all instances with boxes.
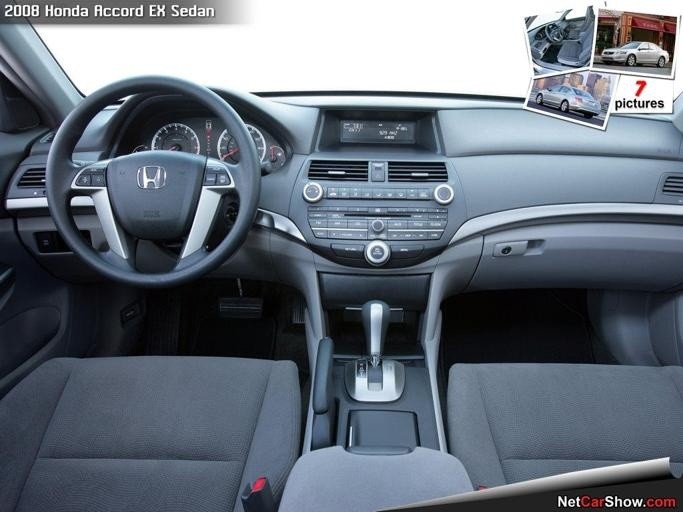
[600,41,669,68]
[534,85,601,119]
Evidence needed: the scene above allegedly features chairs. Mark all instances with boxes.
[1,356,682,512]
